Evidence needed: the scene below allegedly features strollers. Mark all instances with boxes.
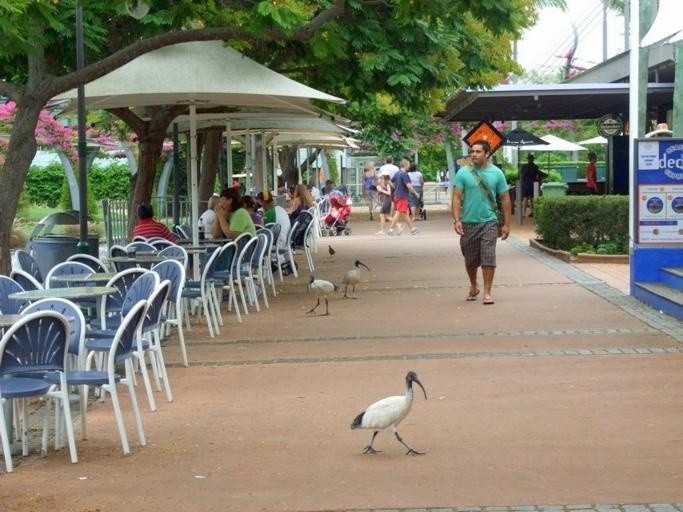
[408,189,426,220]
[319,183,352,237]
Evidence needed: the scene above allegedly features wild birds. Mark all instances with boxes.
[339,259,370,298]
[302,274,338,317]
[349,370,426,457]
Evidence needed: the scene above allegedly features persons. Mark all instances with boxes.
[586,152,601,196]
[521,154,539,217]
[361,156,425,236]
[452,140,511,306]
[132,203,177,247]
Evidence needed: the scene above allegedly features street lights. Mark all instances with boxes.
[71,1,154,261]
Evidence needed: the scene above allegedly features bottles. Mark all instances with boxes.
[197,217,205,242]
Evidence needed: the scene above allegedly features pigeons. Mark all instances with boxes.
[328,245,335,256]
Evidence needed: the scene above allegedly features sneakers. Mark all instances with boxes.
[246,286,262,302]
[375,223,421,236]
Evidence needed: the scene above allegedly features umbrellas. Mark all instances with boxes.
[501,125,550,146]
[578,136,614,145]
[518,134,589,174]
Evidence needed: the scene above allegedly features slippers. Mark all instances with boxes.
[466,288,479,301]
[482,297,494,304]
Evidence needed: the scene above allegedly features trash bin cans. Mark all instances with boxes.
[541,182,569,196]
[31,210,99,289]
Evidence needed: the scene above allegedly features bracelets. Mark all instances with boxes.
[454,220,459,224]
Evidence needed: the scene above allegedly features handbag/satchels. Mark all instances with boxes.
[495,209,503,237]
